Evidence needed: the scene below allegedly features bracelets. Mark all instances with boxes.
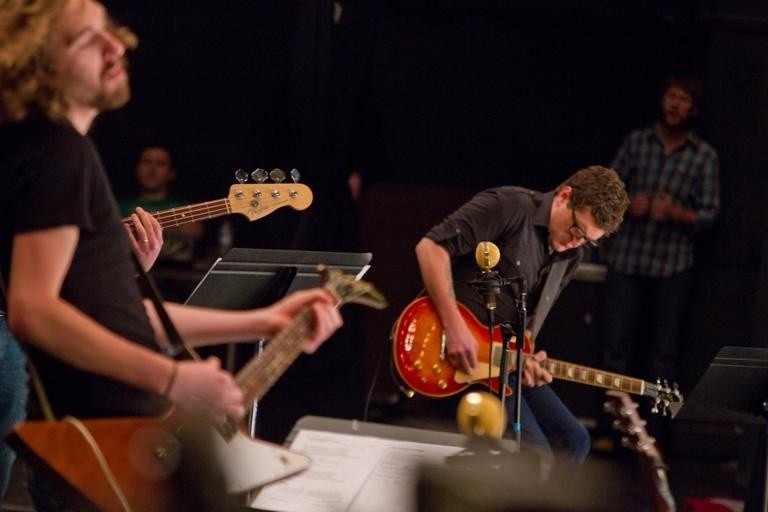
[163,360,178,400]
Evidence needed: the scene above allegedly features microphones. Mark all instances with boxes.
[456,390,509,439]
[474,241,501,270]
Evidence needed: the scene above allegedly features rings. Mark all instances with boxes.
[140,239,148,243]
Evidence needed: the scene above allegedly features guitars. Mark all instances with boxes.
[123,168,313,239]
[389,296,684,419]
[5,263,388,511]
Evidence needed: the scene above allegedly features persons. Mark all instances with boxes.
[588,78,720,457]
[118,139,203,307]
[1,1,344,512]
[415,165,631,481]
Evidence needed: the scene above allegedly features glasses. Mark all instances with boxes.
[568,206,604,252]
